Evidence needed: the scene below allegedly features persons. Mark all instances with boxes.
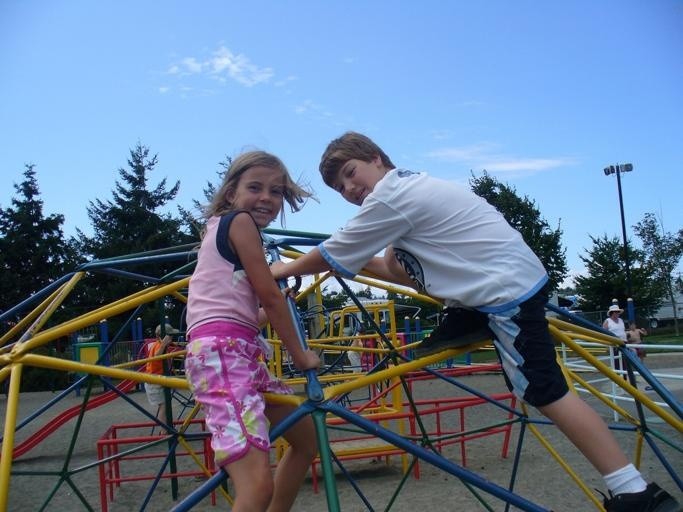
[347,327,364,374]
[184,144,323,511]
[625,321,648,363]
[143,322,181,436]
[602,304,630,387]
[269,128,683,512]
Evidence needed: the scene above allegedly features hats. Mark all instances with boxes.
[606,304,625,317]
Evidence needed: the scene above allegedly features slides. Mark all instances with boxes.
[0,364,147,459]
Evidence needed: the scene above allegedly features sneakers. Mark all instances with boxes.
[594,476,683,512]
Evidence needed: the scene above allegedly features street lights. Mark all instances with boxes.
[604,163,635,322]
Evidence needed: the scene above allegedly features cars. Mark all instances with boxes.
[649,303,683,329]
